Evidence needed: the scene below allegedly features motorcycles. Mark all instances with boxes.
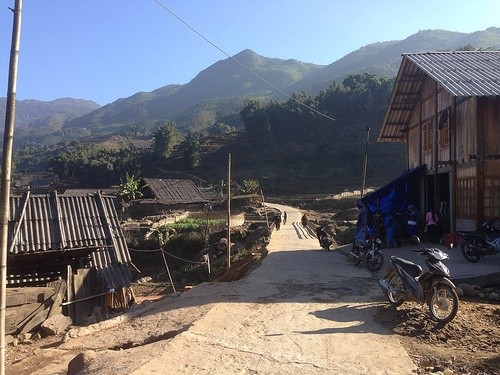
[379,235,459,324]
[349,229,385,272]
[457,216,500,263]
[315,224,335,252]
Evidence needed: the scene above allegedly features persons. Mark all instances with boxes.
[425,205,440,243]
[283,210,287,224]
[275,213,281,231]
[302,213,308,227]
[375,205,420,249]
